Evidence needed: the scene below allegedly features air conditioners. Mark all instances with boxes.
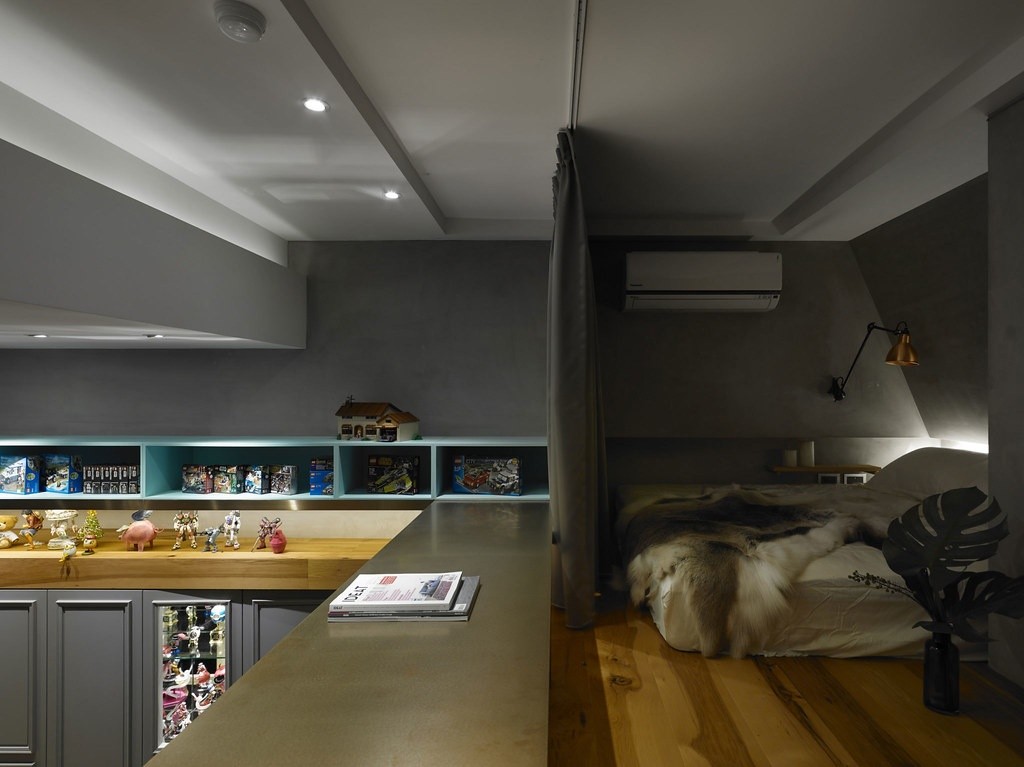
[621,251,782,312]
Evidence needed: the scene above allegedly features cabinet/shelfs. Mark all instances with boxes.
[143,589,336,765]
[0,590,141,767]
[0,434,551,502]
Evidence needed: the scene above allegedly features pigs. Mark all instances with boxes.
[117,521,164,552]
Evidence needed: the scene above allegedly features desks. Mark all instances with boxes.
[764,462,882,474]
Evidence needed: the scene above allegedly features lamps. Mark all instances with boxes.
[827,320,919,402]
[210,0,266,44]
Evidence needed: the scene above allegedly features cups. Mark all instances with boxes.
[784,448,797,467]
[800,441,815,466]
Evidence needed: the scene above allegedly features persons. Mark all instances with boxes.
[171,511,198,550]
[256,517,281,549]
[200,527,220,553]
[223,511,241,549]
[19,508,42,548]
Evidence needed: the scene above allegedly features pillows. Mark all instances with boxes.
[863,447,988,501]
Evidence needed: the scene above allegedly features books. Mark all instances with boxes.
[327,571,481,621]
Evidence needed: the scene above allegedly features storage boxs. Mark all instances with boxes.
[310,458,334,495]
[0,456,139,494]
[181,464,297,495]
[452,455,525,496]
[367,455,420,494]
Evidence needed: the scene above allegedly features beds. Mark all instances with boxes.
[607,437,988,660]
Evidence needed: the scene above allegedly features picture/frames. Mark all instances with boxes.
[843,473,867,484]
[818,472,840,484]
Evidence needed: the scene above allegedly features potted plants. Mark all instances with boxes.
[847,487,1024,715]
